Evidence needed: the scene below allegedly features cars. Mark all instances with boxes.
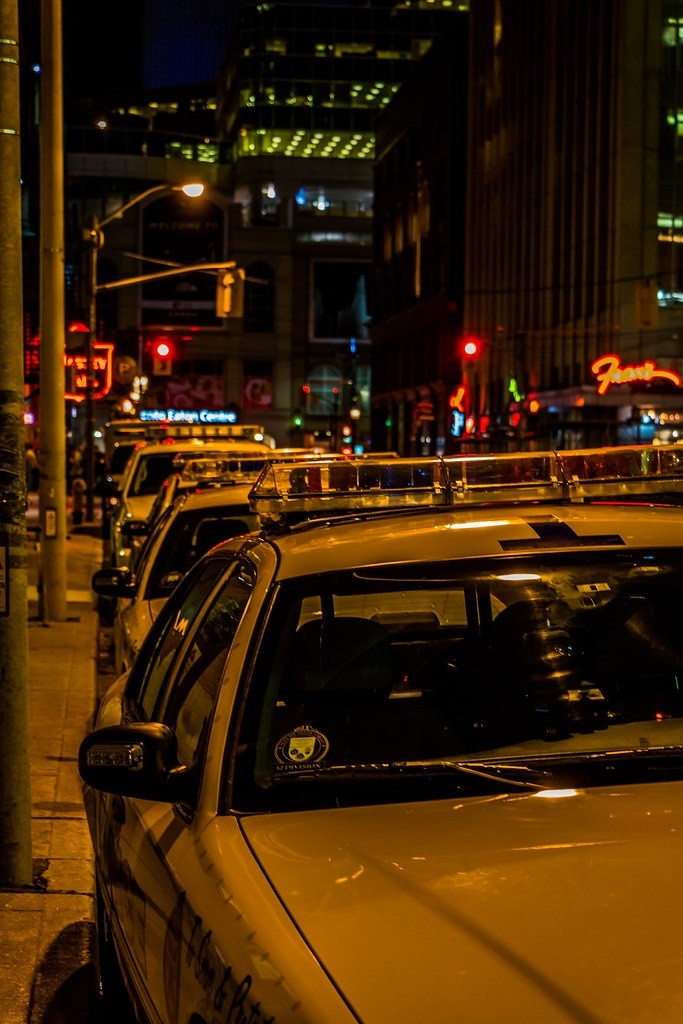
[94,441,362,683]
[79,500,682,1017]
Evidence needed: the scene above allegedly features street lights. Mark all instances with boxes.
[75,180,213,605]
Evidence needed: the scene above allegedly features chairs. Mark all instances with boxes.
[282,610,427,769]
